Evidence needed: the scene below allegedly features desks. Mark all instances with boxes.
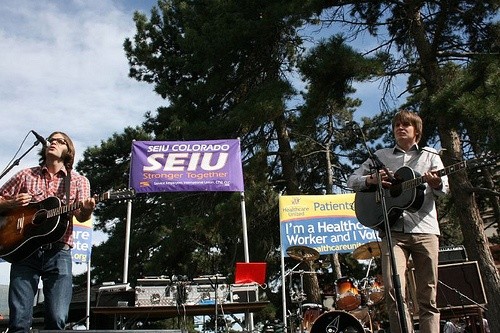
[440,306,486,333]
[91,302,270,333]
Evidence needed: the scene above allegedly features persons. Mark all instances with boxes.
[0,132,98,333]
[347,109,449,333]
[442,316,490,333]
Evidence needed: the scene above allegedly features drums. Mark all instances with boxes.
[310,310,367,333]
[301,301,322,333]
[333,277,361,309]
[364,282,386,304]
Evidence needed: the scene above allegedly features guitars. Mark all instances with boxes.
[355,152,494,228]
[1,187,136,262]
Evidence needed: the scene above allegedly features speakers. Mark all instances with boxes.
[408,261,488,313]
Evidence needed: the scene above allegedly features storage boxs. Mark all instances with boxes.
[411,261,488,314]
[230,283,259,303]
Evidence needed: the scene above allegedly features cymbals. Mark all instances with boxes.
[352,241,383,257]
[287,245,317,259]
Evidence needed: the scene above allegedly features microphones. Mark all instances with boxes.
[32,130,51,148]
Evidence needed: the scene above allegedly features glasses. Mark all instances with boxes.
[47,138,68,145]
